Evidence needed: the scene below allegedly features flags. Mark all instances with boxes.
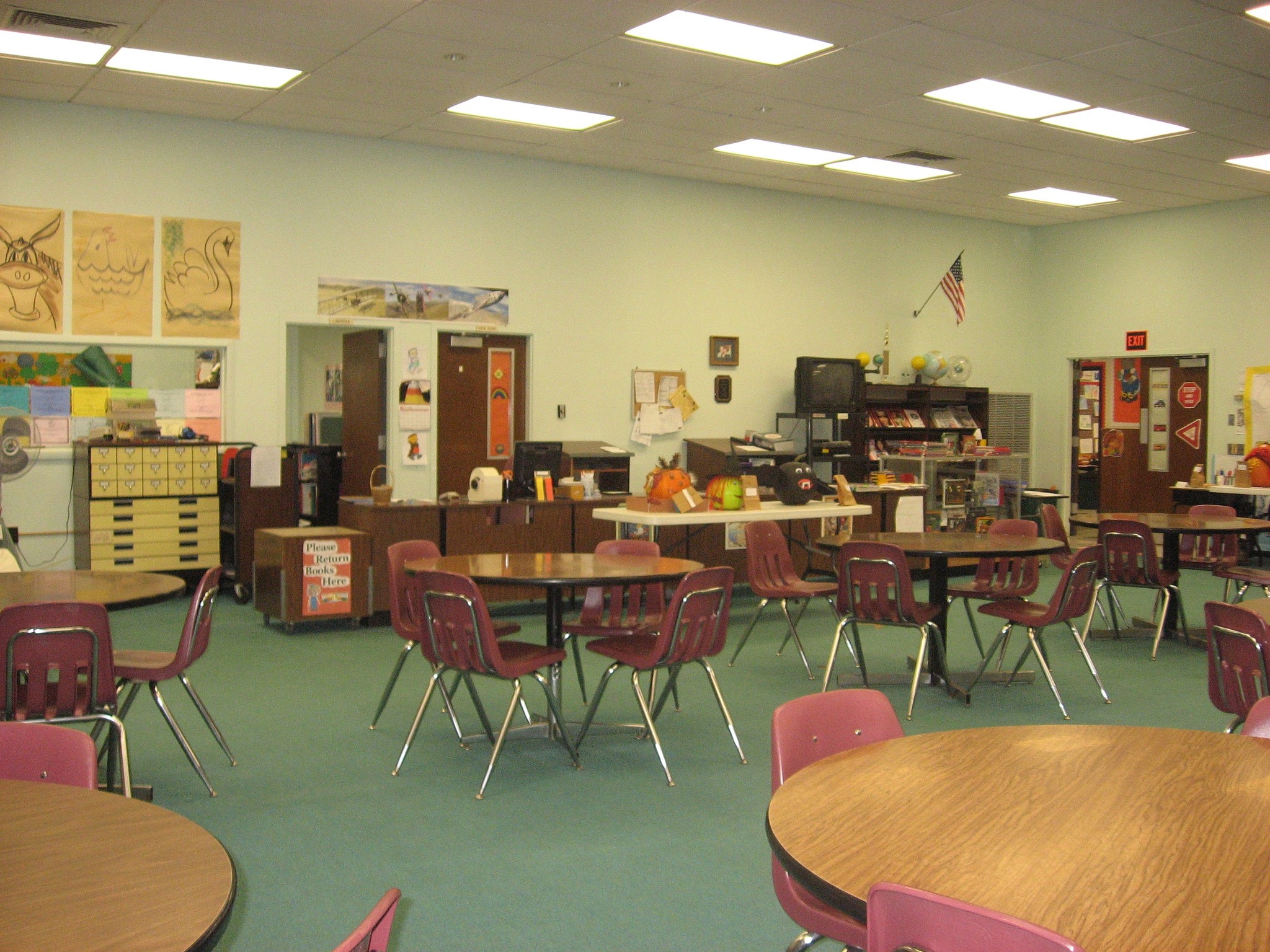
[939,255,966,327]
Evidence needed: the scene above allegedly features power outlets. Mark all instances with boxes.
[8,527,19,545]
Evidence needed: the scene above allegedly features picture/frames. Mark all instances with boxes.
[709,335,739,366]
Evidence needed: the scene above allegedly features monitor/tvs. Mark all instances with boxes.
[508,442,562,499]
[795,356,866,413]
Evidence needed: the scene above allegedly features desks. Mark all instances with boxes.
[767,727,1270,952]
[1170,486,1264,566]
[397,549,700,754]
[1069,508,1270,655]
[814,528,1066,701]
[0,778,240,952]
[7,567,187,796]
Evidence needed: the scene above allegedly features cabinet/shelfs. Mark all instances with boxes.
[253,487,929,631]
[776,412,865,483]
[281,443,341,525]
[866,383,988,485]
[71,440,221,595]
[551,440,633,492]
[218,442,258,603]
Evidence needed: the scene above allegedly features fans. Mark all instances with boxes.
[0,406,42,518]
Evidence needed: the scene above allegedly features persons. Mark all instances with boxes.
[877,439,887,457]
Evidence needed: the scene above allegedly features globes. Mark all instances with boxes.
[923,351,949,385]
[873,354,885,373]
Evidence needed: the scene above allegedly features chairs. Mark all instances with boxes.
[0,564,242,797]
[333,502,1270,951]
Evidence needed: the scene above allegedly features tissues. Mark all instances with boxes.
[558,476,584,501]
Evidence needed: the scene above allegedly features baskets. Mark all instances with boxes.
[370,466,394,505]
